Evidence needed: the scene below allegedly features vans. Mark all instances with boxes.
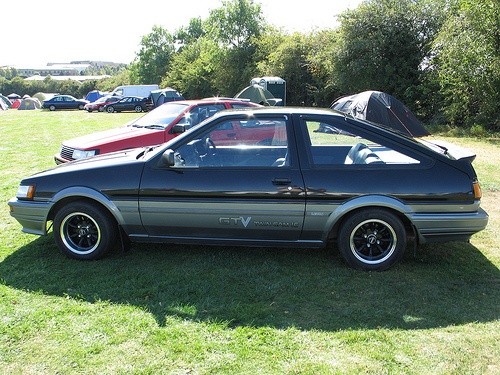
[111,84,159,100]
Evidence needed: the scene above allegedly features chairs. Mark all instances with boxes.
[344,143,385,164]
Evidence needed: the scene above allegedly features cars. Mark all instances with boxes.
[103,96,154,113]
[42,95,91,111]
[8,106,490,271]
[53,96,287,174]
[83,95,122,112]
